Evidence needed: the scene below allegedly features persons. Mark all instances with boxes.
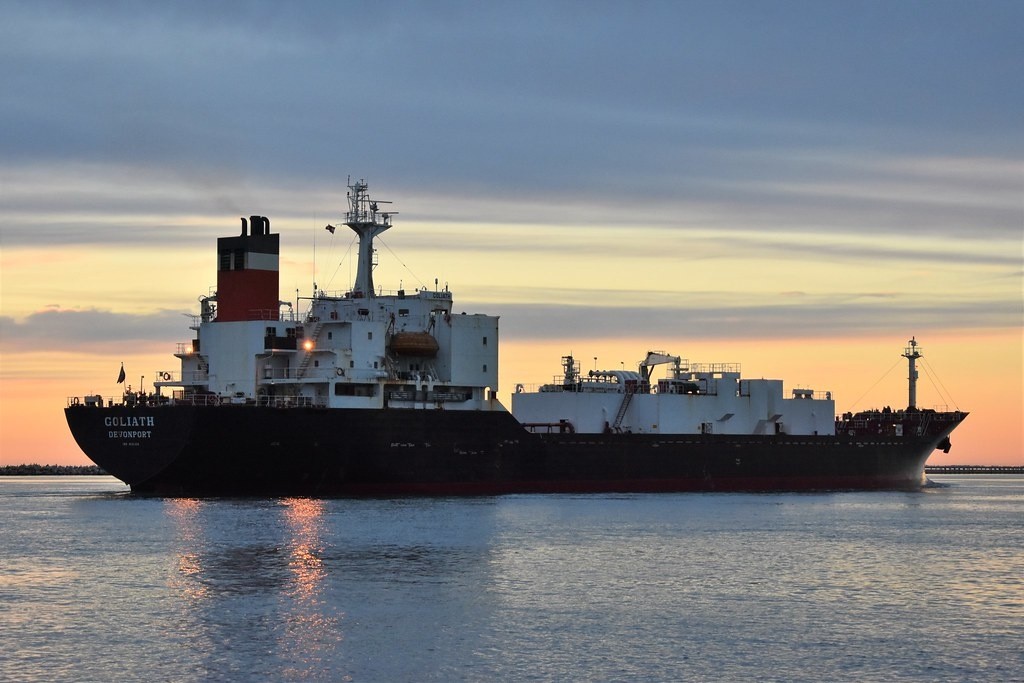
[127,385,131,391]
[133,391,153,406]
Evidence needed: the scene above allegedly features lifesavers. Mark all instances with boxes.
[162,372,170,381]
[337,368,343,376]
[515,383,524,393]
[73,397,79,405]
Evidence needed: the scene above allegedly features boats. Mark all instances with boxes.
[64,173,970,501]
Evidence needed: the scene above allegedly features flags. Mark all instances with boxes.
[326,223,335,234]
[116,366,125,384]
